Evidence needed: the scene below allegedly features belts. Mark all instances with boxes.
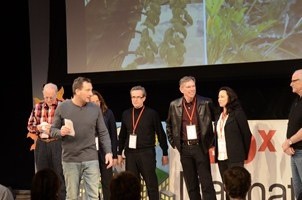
[181,140,199,145]
[38,138,58,142]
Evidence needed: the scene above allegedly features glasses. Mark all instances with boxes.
[131,96,144,99]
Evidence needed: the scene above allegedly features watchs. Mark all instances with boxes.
[288,138,293,145]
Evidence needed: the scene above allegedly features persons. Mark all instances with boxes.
[109,170,141,200]
[27,82,67,200]
[88,89,118,200]
[166,76,216,200]
[222,164,252,200]
[214,86,252,200]
[49,77,115,200]
[281,68,302,200]
[117,86,169,200]
[30,168,65,200]
